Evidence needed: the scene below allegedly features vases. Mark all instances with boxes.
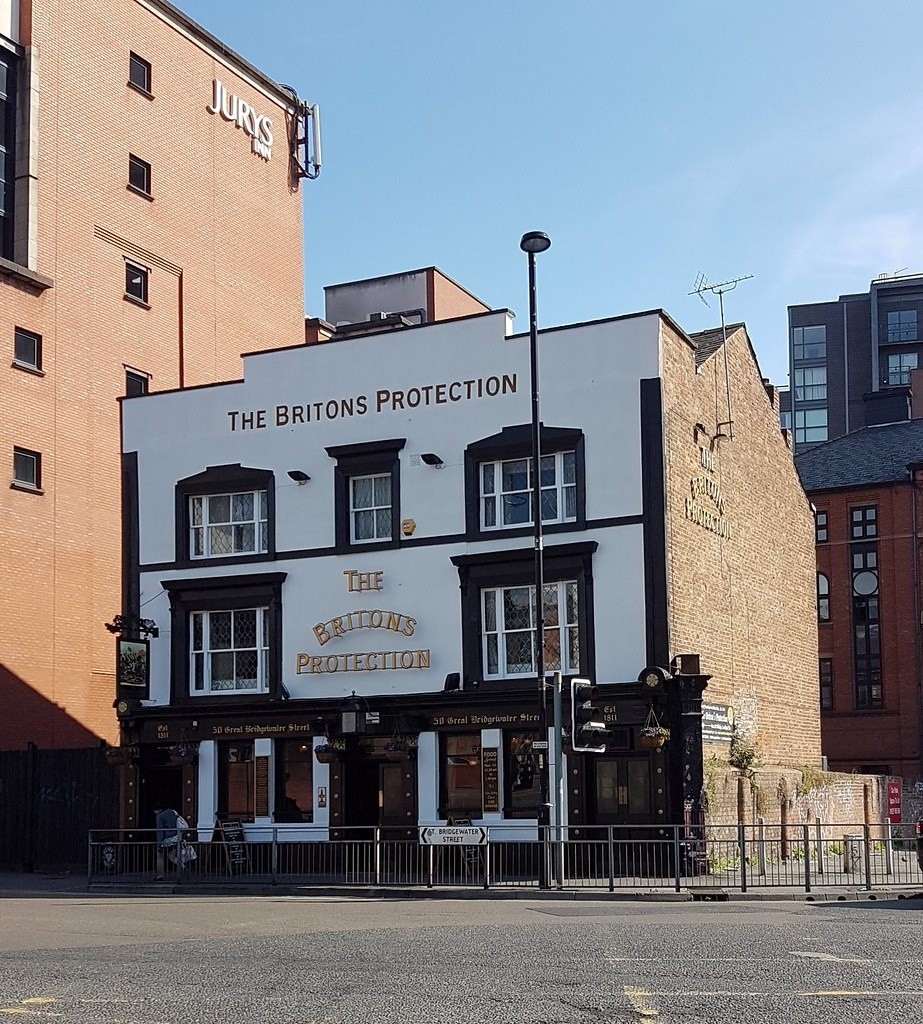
[640,737,665,747]
[170,755,195,766]
[386,751,409,763]
[315,750,337,764]
[106,757,129,766]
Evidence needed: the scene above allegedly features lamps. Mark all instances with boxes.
[420,453,445,469]
[339,690,402,737]
[277,684,291,701]
[441,673,461,693]
[287,471,312,485]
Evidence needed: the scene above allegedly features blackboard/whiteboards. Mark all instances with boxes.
[221,823,251,860]
[446,815,485,868]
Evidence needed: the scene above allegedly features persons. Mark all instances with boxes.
[152,802,188,881]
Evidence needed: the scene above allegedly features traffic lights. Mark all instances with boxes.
[570,678,615,753]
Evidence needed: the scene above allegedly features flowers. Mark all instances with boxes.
[314,744,333,751]
[167,743,200,758]
[639,727,672,741]
[383,739,410,751]
[105,747,131,758]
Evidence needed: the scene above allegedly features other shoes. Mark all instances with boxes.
[153,877,164,881]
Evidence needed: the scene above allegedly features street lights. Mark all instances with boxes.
[519,229,557,894]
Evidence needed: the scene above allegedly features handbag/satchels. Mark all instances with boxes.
[181,840,197,864]
[172,810,189,829]
